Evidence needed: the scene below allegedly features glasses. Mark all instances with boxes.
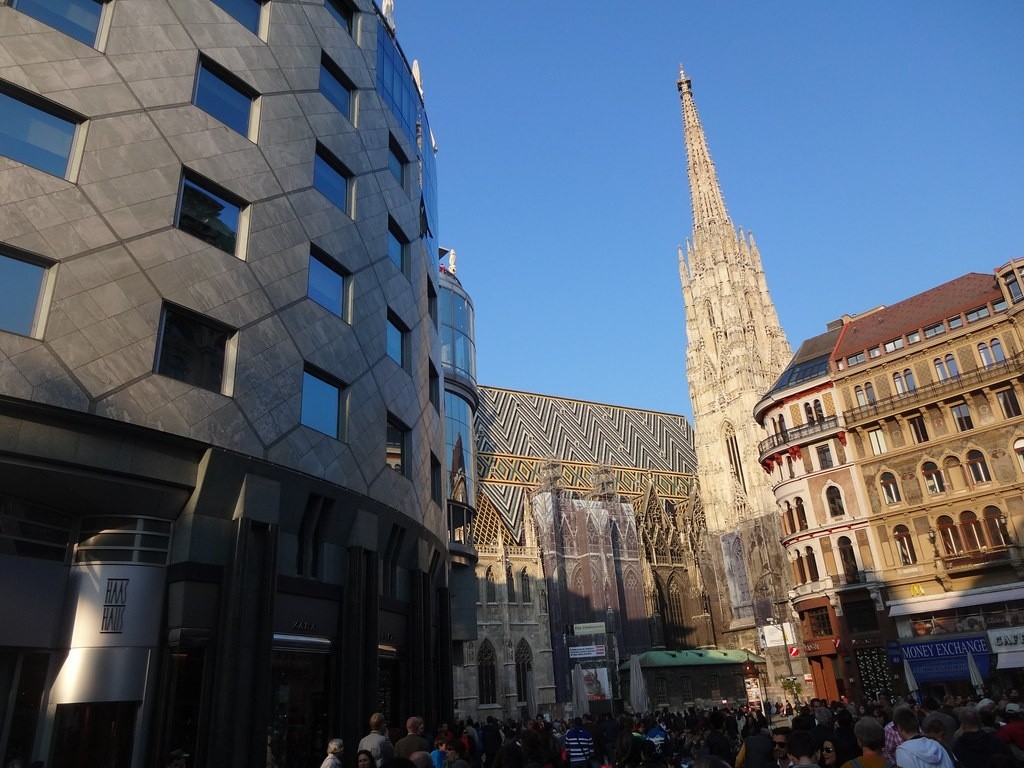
[872,715,882,719]
[892,725,899,731]
[771,740,788,749]
[445,747,454,751]
[820,746,835,754]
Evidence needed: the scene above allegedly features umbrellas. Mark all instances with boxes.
[629,655,648,714]
[526,671,539,719]
[572,664,590,720]
[903,660,920,705]
[967,652,984,695]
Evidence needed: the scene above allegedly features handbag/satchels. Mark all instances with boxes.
[587,757,600,768]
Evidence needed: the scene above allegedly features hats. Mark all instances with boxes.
[1006,703,1021,713]
[974,698,995,712]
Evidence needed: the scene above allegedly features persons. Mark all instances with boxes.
[319,691,1024,768]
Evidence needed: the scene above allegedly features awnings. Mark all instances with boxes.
[616,648,765,667]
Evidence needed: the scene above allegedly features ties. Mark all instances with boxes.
[540,724,544,730]
